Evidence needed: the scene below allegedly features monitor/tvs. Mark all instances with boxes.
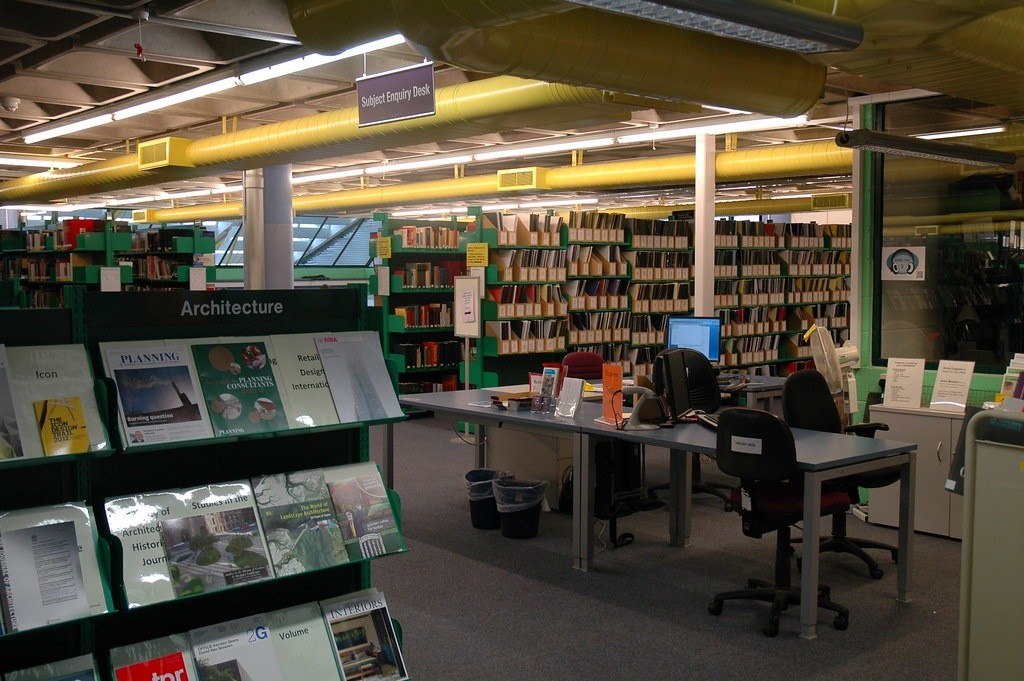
[652,350,693,421]
[666,316,722,363]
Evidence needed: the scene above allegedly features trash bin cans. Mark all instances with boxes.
[491,480,546,540]
[466,470,513,529]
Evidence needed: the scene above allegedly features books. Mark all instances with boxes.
[5,218,216,308]
[393,211,852,394]
[1000,353,1024,400]
[0,329,408,681]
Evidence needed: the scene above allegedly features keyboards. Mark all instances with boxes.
[697,414,719,429]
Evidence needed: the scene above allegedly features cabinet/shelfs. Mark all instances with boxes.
[455,206,851,435]
[0,225,217,307]
[0,281,412,681]
[867,403,965,540]
[368,212,476,419]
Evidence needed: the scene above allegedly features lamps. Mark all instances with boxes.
[21,32,407,144]
[946,308,982,353]
[0,111,811,212]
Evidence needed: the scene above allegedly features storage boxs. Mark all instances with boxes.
[62,219,95,249]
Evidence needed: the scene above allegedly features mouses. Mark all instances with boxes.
[693,410,705,414]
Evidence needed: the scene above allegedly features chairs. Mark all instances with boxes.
[781,368,899,579]
[706,406,850,637]
[648,347,734,513]
[562,351,604,379]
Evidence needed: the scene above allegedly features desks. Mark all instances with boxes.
[397,376,918,639]
